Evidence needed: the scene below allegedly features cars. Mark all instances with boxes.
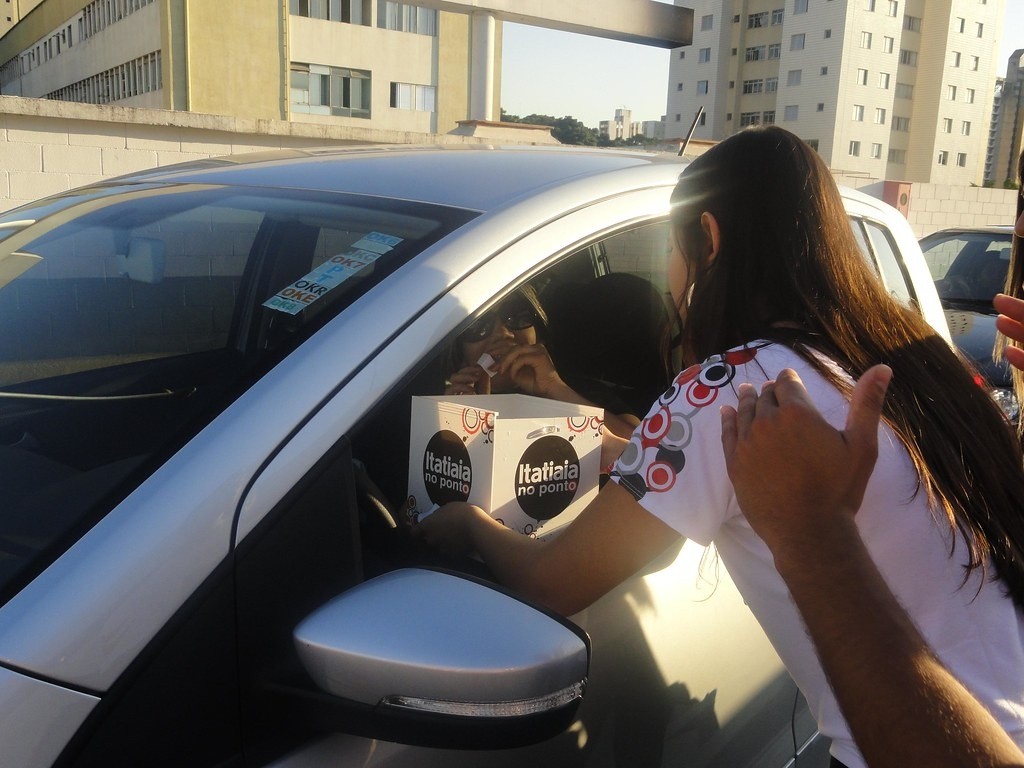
[1,103,956,768]
[914,224,1024,453]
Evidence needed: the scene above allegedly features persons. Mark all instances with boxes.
[412,126,1024,767]
[992,149,1024,428]
[722,292,1024,767]
[442,280,635,471]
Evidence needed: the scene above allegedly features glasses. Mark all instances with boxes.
[465,300,536,344]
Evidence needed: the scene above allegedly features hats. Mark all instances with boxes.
[529,272,675,415]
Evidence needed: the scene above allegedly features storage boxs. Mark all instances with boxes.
[403,393,605,545]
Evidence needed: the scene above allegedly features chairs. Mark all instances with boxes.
[545,271,683,423]
